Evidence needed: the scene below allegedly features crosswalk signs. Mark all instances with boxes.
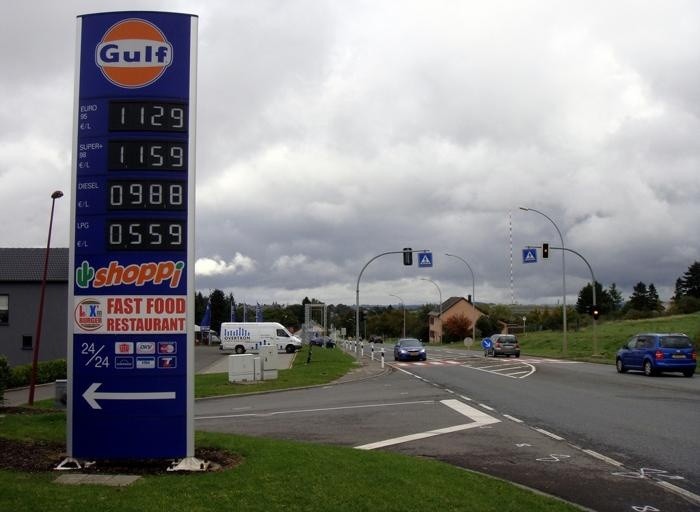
[418,252,433,267]
[522,248,537,263]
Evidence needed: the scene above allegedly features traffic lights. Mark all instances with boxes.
[593,305,599,320]
[543,243,548,258]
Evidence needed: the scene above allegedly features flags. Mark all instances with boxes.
[257,302,264,322]
[200,295,210,326]
[244,302,249,323]
[231,301,239,322]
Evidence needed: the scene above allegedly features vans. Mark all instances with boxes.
[219,322,303,353]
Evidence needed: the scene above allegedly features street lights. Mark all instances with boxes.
[444,254,475,345]
[519,206,568,357]
[420,278,443,345]
[390,295,406,338]
[29,191,64,405]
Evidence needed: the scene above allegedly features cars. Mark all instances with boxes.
[310,335,336,349]
[485,334,520,359]
[394,338,426,362]
[356,335,384,343]
[616,333,697,378]
